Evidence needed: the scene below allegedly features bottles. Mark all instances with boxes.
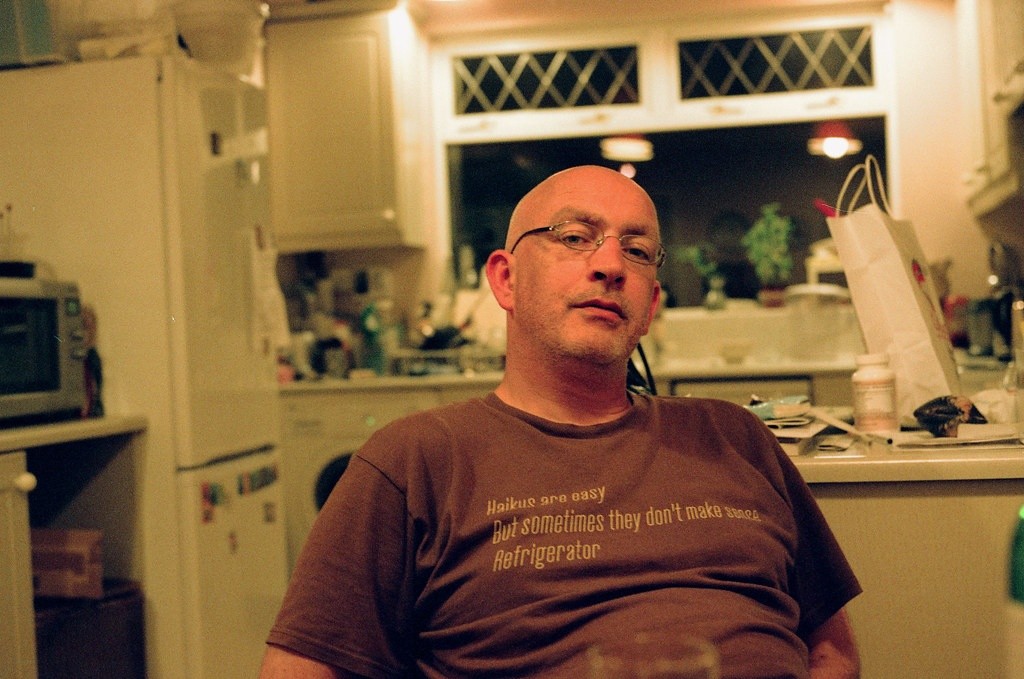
[945,294,993,355]
[850,352,900,438]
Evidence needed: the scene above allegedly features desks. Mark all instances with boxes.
[772,436,1024,679]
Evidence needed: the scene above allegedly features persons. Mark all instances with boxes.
[261,164,864,679]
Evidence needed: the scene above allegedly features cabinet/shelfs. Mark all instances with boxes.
[669,373,819,412]
[262,12,426,253]
[0,416,146,679]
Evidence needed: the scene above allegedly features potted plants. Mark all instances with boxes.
[674,241,726,310]
[739,203,793,305]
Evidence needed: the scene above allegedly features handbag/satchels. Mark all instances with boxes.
[826,154,961,428]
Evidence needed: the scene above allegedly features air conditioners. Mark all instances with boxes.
[808,121,862,158]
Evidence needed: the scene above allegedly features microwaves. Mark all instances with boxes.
[0,276,88,421]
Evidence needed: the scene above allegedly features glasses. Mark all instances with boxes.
[511,220,667,272]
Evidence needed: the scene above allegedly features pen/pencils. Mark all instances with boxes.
[865,433,892,444]
